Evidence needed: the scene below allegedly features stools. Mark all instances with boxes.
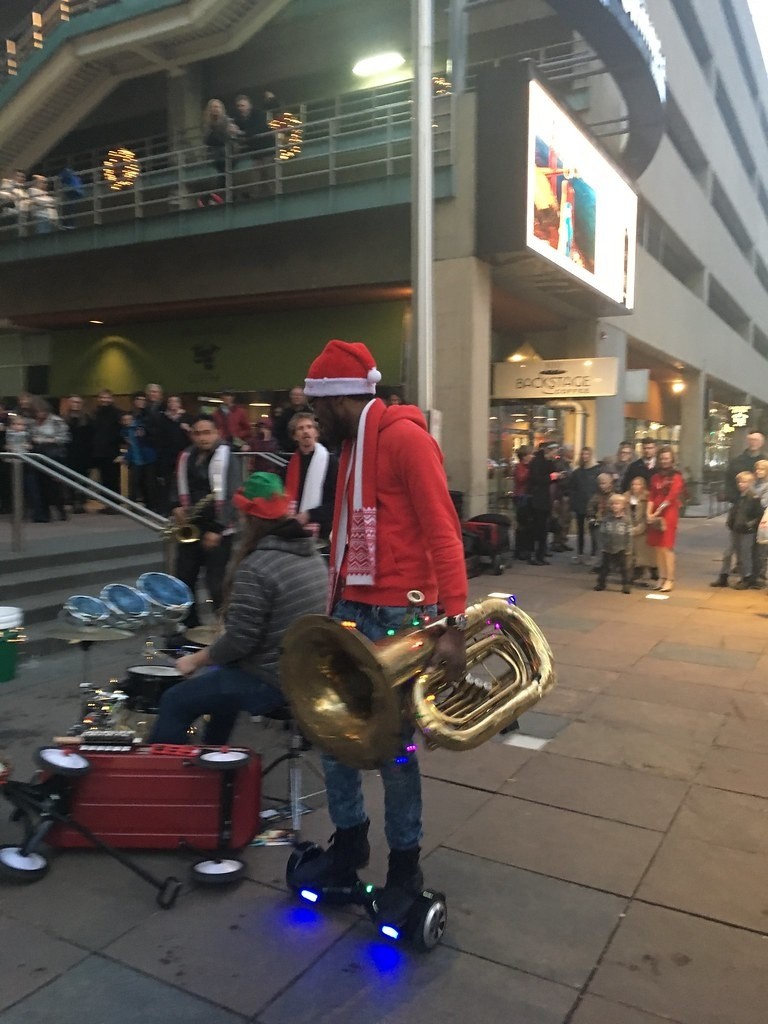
[259,703,325,842]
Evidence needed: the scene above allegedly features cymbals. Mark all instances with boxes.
[45,625,135,642]
[180,625,227,646]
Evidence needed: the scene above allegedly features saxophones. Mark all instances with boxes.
[158,483,216,546]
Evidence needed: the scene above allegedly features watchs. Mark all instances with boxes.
[446,614,470,630]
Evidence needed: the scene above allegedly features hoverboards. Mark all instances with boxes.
[284,840,448,952]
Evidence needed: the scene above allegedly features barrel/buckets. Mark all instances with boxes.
[0,607,23,683]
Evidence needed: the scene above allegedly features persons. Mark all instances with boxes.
[388,390,401,405]
[276,413,339,566]
[592,494,635,594]
[291,339,466,930]
[144,382,166,416]
[87,389,129,514]
[0,391,71,519]
[117,411,157,503]
[131,391,159,443]
[58,389,97,513]
[620,476,658,587]
[155,395,194,518]
[163,413,241,628]
[513,431,768,590]
[642,445,681,592]
[210,389,306,475]
[0,169,58,238]
[142,471,330,750]
[195,91,274,208]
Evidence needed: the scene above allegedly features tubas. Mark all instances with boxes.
[277,587,559,771]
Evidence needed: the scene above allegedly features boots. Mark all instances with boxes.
[709,573,729,587]
[539,550,550,565]
[378,847,425,929]
[290,816,372,889]
[734,575,755,590]
[527,551,545,566]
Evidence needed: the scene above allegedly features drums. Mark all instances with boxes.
[100,583,152,630]
[125,664,186,714]
[63,594,109,628]
[136,571,195,623]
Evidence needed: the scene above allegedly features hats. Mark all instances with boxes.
[303,338,382,397]
[231,471,292,520]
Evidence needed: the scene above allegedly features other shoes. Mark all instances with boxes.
[622,587,630,594]
[594,585,606,592]
[652,577,666,590]
[660,579,676,592]
